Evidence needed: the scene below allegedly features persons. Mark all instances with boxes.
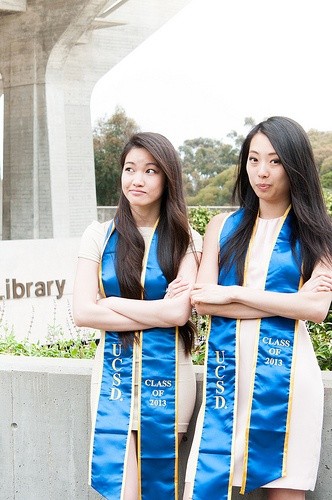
[188,116,332,500]
[71,131,202,498]
